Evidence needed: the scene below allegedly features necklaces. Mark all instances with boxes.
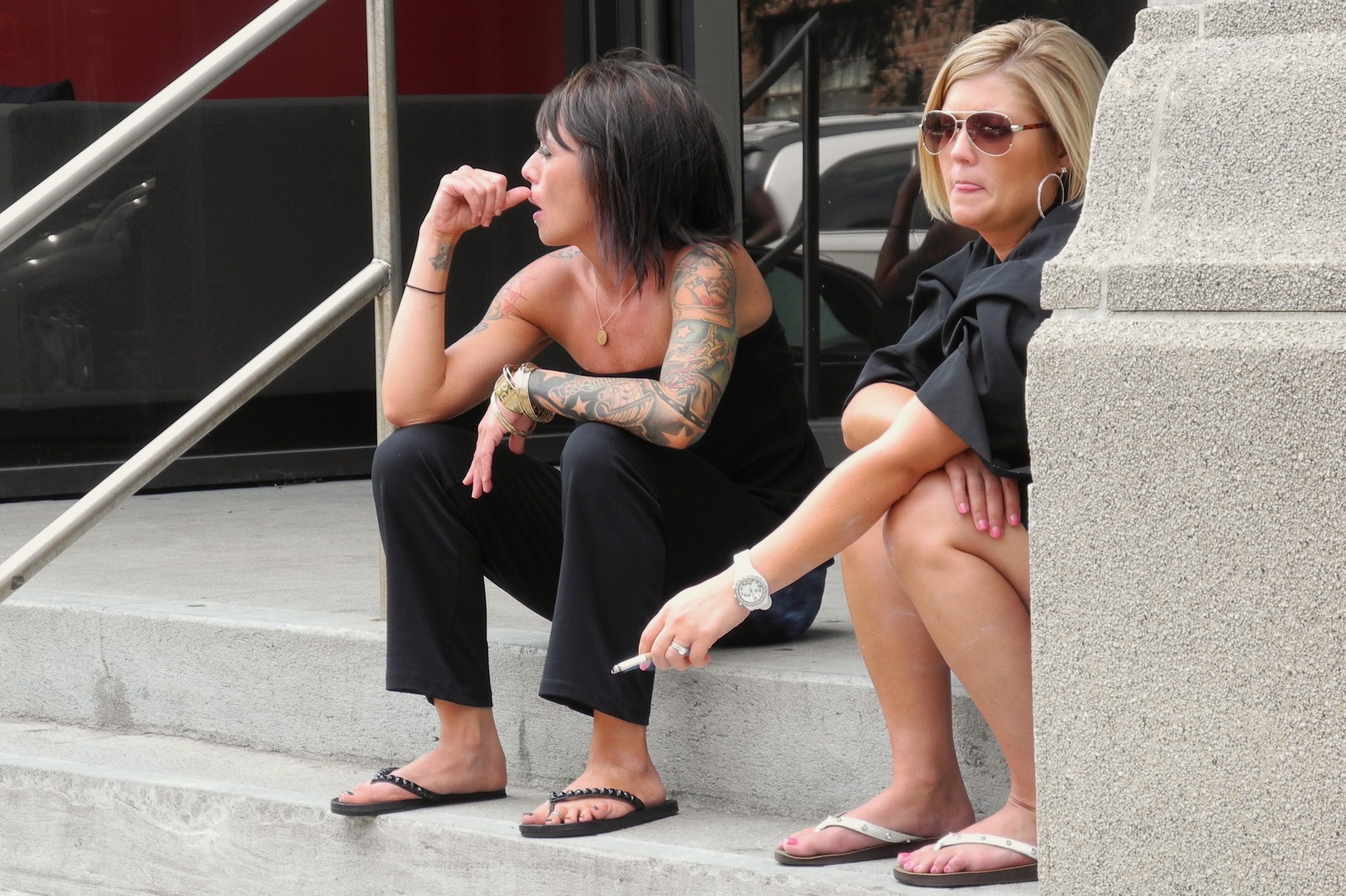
[595,251,651,346]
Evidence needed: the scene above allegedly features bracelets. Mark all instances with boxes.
[493,374,524,414]
[490,391,536,437]
[515,362,555,423]
[502,364,519,389]
[404,282,448,294]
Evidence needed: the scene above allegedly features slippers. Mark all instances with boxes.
[773,811,941,866]
[330,767,507,817]
[893,829,1039,887]
[518,786,680,839]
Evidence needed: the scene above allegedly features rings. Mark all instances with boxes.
[670,641,689,656]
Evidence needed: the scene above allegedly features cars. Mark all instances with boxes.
[745,113,935,287]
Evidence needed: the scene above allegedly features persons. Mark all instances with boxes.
[333,45,834,839]
[744,173,784,247]
[639,18,1108,888]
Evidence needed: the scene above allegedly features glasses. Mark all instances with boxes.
[918,110,1051,157]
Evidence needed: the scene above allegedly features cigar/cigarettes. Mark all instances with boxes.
[611,650,653,674]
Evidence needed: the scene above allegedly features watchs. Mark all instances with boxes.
[730,549,773,610]
